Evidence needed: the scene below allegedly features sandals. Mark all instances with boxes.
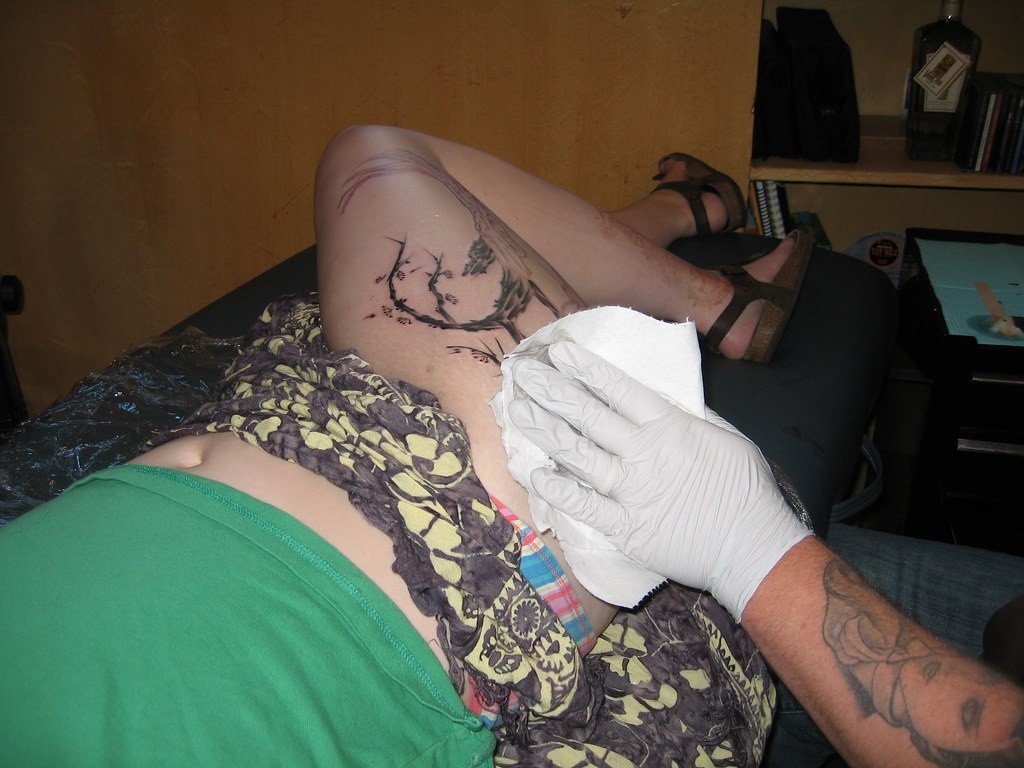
[703,229,813,365]
[650,153,746,238]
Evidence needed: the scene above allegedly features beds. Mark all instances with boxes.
[1,241,893,768]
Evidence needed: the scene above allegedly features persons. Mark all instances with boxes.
[507,341,1024,768]
[0,116,816,768]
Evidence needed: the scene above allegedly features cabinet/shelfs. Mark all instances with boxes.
[859,228,1023,561]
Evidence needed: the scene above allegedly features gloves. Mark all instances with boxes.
[506,340,816,623]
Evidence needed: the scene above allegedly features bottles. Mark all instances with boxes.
[904,1,982,161]
